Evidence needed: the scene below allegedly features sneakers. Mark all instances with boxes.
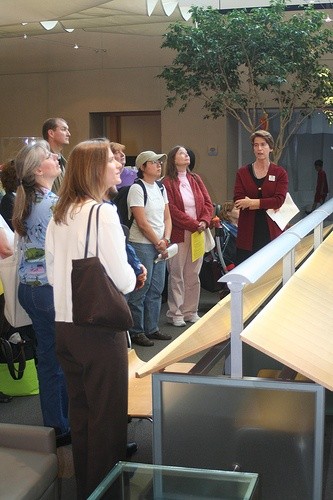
[184,314,201,322]
[146,330,172,340]
[168,318,186,326]
[130,332,154,346]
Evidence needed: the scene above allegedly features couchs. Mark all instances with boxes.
[0,423,61,500]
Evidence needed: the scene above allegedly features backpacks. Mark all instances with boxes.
[113,178,164,240]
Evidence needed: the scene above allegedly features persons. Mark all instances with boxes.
[233,129,288,266]
[305,159,328,215]
[0,118,140,402]
[13,140,72,449]
[160,145,213,326]
[127,151,172,347]
[220,200,240,227]
[44,137,136,500]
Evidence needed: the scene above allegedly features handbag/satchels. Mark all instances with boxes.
[0,325,34,380]
[0,358,39,403]
[0,293,35,342]
[204,227,216,252]
[199,252,227,292]
[71,203,134,329]
[0,230,32,328]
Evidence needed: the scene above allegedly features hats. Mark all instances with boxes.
[135,151,167,170]
[117,167,137,188]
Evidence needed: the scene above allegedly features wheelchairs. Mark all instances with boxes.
[213,203,238,300]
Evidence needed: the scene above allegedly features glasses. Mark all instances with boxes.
[146,161,164,165]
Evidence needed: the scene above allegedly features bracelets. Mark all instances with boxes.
[163,237,170,248]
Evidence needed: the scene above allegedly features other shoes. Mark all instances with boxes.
[127,443,138,452]
[56,435,72,447]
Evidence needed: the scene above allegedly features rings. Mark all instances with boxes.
[201,230,203,232]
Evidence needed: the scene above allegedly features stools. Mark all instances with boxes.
[127,348,197,462]
